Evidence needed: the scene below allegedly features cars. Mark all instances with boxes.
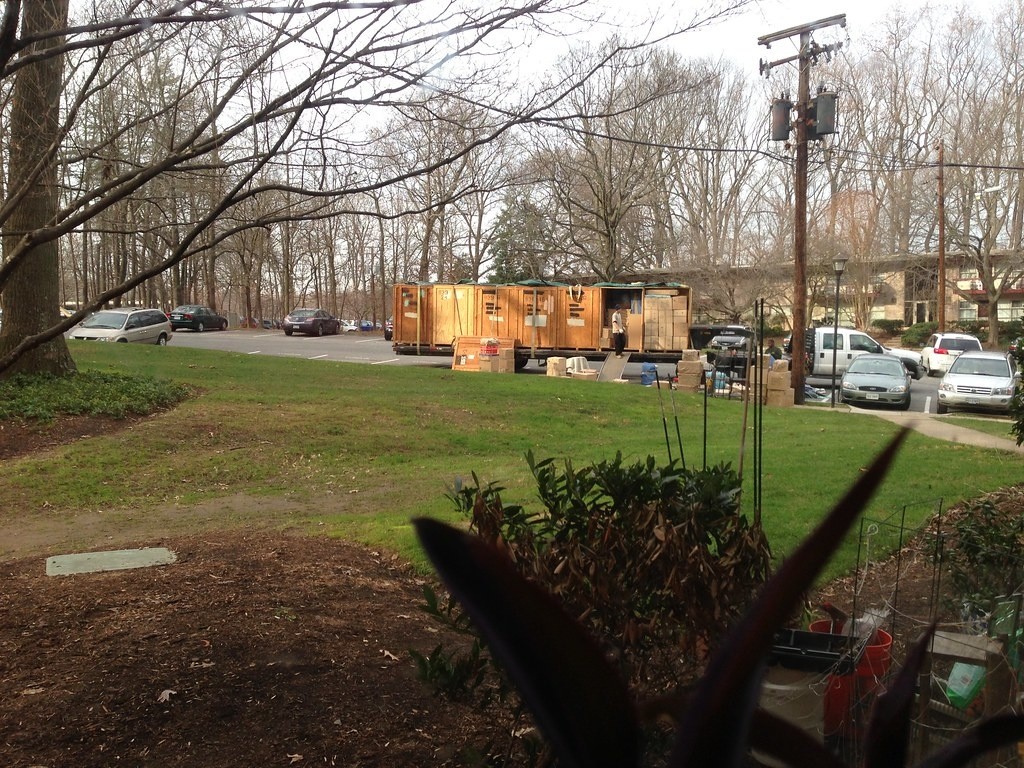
[937,350,1021,413]
[68,306,173,346]
[837,353,916,411]
[239,316,285,330]
[165,304,228,332]
[384,315,393,341]
[1009,336,1024,360]
[336,317,384,332]
[781,333,792,350]
[707,335,753,367]
[284,308,340,337]
[60,301,83,319]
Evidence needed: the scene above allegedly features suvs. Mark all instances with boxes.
[918,333,984,377]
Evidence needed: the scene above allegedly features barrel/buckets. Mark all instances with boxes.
[742,661,826,768]
[809,617,893,749]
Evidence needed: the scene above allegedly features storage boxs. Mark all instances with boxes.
[773,360,788,372]
[545,356,567,377]
[479,345,498,353]
[757,353,770,368]
[678,360,703,373]
[749,393,765,405]
[499,359,515,373]
[641,362,672,390]
[767,388,794,407]
[478,354,499,372]
[767,371,791,389]
[500,348,514,358]
[677,373,701,386]
[749,365,771,384]
[600,309,628,349]
[644,289,689,349]
[682,350,700,361]
[750,384,767,396]
[572,373,595,380]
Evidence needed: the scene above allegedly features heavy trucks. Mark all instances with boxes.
[391,278,757,379]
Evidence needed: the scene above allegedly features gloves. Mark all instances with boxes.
[619,329,624,333]
[622,327,626,332]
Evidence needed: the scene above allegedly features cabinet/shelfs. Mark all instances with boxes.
[914,630,1009,768]
[627,314,641,348]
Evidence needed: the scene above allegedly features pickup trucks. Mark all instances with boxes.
[806,327,924,387]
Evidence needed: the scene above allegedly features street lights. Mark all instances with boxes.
[770,80,837,409]
[831,252,849,409]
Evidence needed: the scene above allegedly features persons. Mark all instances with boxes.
[611,304,626,358]
[765,339,782,360]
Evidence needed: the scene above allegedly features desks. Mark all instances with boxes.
[725,378,749,401]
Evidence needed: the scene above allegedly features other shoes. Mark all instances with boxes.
[616,355,622,358]
[620,353,624,356]
